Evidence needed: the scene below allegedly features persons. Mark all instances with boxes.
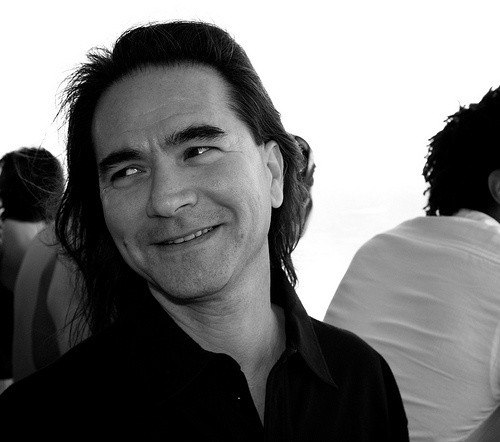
[323,84,500,442]
[0,148,64,394]
[2,21,410,441]
